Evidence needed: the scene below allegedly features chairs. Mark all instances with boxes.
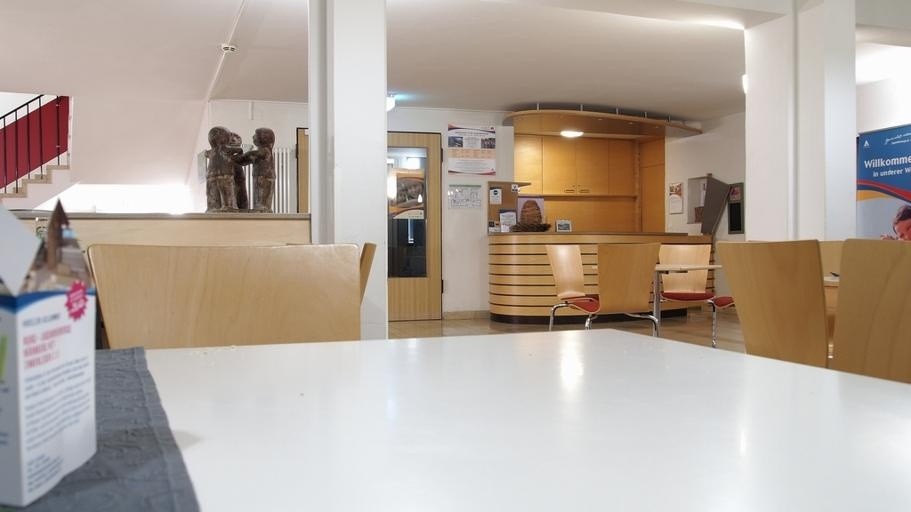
[86,244,362,350]
[716,240,829,369]
[542,244,599,331]
[568,241,661,338]
[659,244,716,347]
[830,243,911,382]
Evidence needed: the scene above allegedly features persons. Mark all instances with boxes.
[879,204,911,245]
[233,127,278,212]
[227,129,252,210]
[202,125,245,208]
[398,180,424,202]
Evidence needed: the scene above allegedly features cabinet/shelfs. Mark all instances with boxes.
[513,134,543,195]
[607,138,638,198]
[541,136,608,196]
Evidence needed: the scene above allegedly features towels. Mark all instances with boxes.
[0,347,199,510]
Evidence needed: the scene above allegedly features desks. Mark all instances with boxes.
[593,263,723,347]
[825,275,839,317]
[1,327,908,511]
